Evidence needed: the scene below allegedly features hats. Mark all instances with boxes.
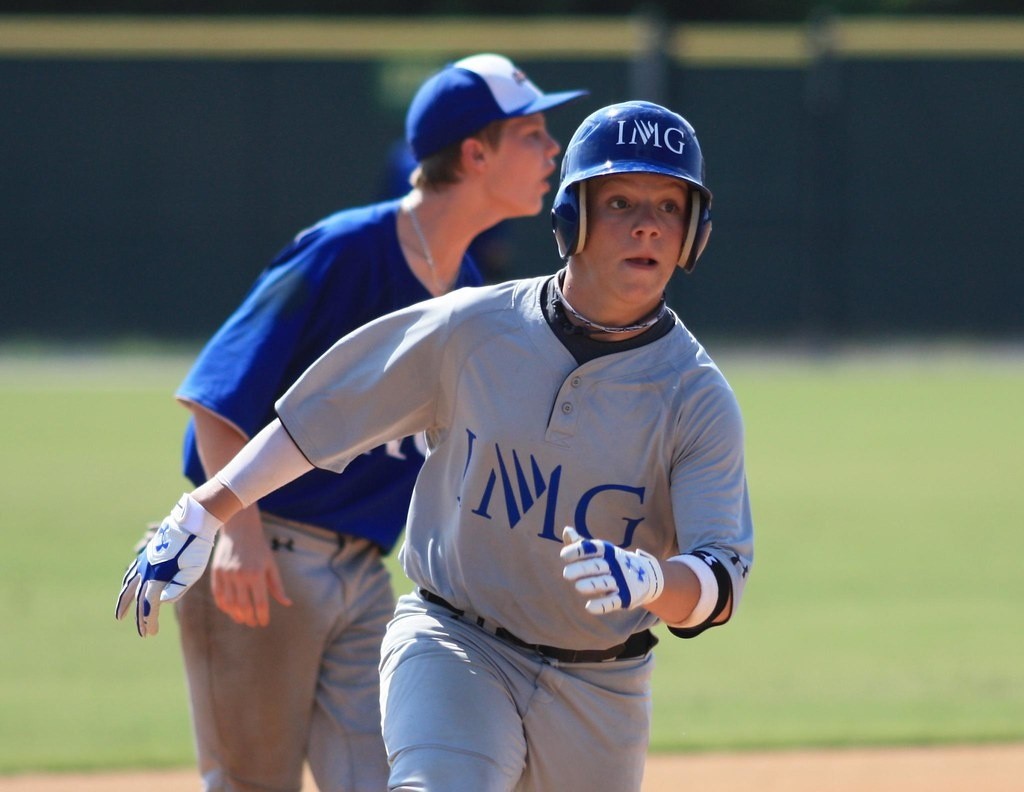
[403,53,589,161]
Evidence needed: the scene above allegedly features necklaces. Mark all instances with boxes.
[406,200,446,291]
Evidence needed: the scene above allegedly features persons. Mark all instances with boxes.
[174,54,586,790]
[115,101,754,792]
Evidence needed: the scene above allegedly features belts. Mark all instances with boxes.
[420,589,649,664]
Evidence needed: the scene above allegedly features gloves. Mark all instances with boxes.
[113,493,225,638]
[559,527,666,614]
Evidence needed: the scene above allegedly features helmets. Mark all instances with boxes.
[551,99,715,273]
